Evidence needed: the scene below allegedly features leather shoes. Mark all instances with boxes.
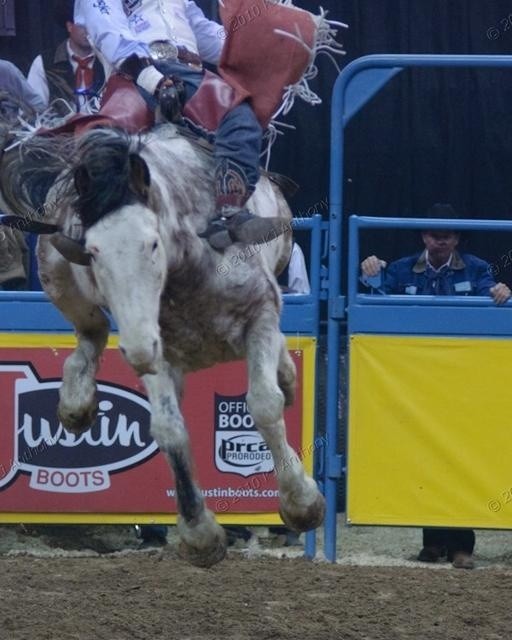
[419,546,446,562]
[447,553,473,568]
[271,533,303,547]
[206,209,294,250]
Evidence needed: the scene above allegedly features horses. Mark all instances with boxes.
[0,116,328,573]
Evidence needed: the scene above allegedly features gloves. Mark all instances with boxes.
[154,77,187,123]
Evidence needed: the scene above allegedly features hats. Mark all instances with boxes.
[425,202,457,218]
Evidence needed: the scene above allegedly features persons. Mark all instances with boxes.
[73,1,290,247]
[361,206,510,568]
[0,16,311,549]
